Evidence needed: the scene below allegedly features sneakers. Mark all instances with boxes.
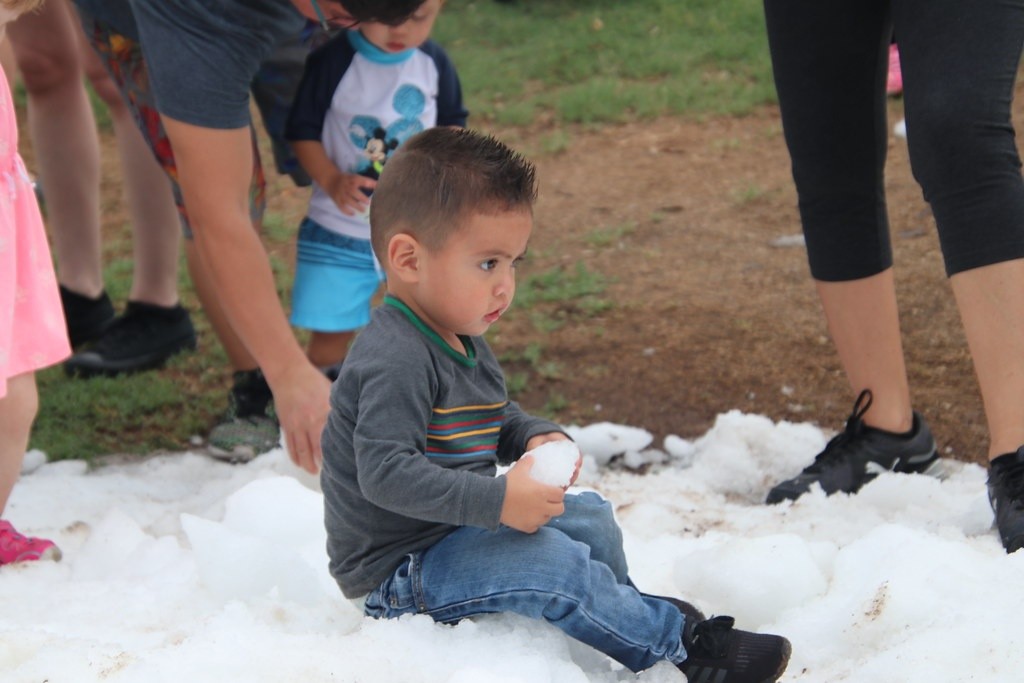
[764,389,946,513]
[62,303,202,377]
[206,370,280,462]
[639,592,707,644]
[0,518,63,564]
[675,614,792,683]
[986,444,1024,553]
[57,284,118,347]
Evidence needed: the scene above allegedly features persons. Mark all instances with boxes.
[0,0,472,564]
[321,126,791,683]
[764,0,1024,555]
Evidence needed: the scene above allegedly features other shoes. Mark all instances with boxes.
[884,42,903,96]
